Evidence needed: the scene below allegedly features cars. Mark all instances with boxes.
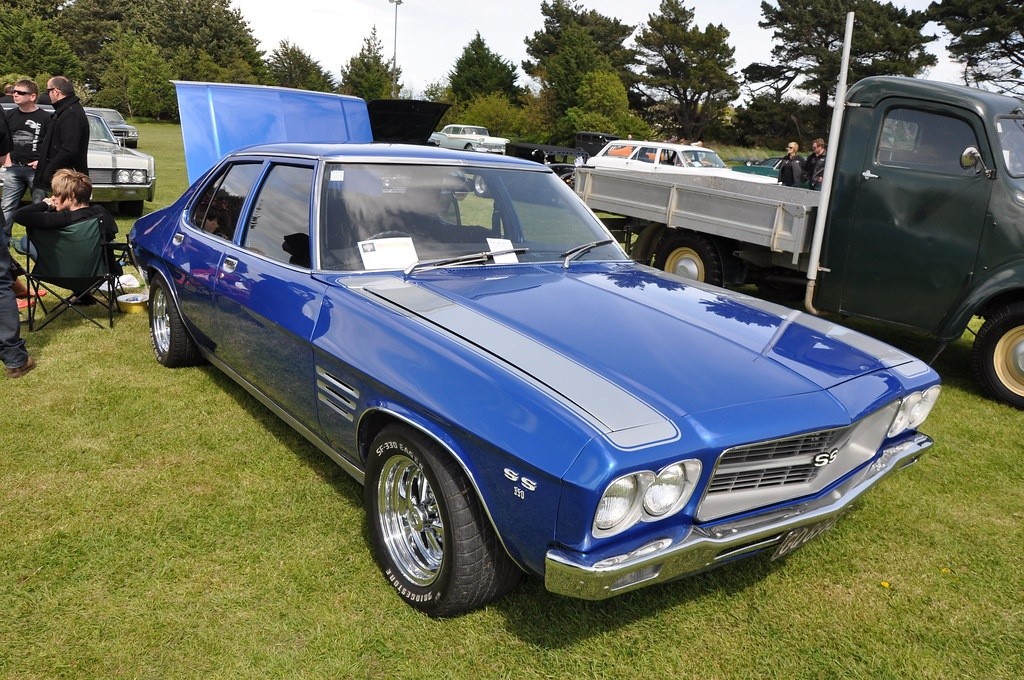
[45,110,157,216]
[427,124,510,154]
[89,106,139,148]
[584,137,780,186]
[128,78,943,626]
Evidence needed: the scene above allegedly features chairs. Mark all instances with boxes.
[24,214,127,329]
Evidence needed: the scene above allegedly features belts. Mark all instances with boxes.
[14,161,27,167]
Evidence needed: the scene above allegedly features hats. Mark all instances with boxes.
[691,141,702,146]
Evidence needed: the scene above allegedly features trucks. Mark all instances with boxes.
[574,75,1024,411]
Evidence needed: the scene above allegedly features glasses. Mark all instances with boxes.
[812,146,818,149]
[788,147,793,149]
[47,88,61,90]
[13,90,34,95]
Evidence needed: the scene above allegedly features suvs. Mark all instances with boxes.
[472,141,589,197]
[734,157,785,178]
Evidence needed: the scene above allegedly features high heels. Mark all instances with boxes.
[16,290,46,299]
[16,298,36,314]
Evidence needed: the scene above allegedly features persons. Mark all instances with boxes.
[0,104,37,378]
[778,138,826,191]
[13,169,119,306]
[2,76,90,249]
[283,233,311,269]
[196,194,232,241]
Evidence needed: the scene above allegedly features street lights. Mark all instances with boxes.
[385,0,405,84]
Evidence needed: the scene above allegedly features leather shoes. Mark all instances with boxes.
[6,357,36,378]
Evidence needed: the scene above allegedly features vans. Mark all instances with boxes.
[574,129,621,157]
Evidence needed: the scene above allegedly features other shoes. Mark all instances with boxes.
[65,295,82,304]
